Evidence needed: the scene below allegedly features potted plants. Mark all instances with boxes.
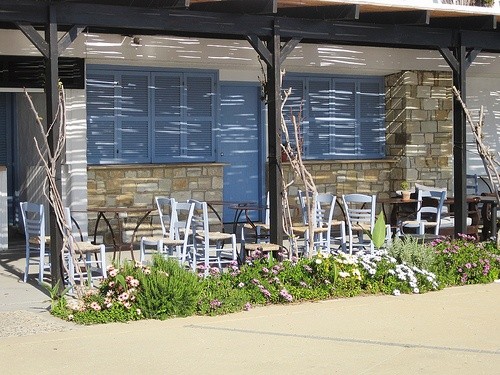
[401,182,410,200]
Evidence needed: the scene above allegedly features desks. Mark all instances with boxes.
[447,195,500,244]
[87,207,158,271]
[372,199,421,237]
[202,201,256,263]
[229,204,299,265]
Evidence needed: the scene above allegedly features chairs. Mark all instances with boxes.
[17,183,500,288]
